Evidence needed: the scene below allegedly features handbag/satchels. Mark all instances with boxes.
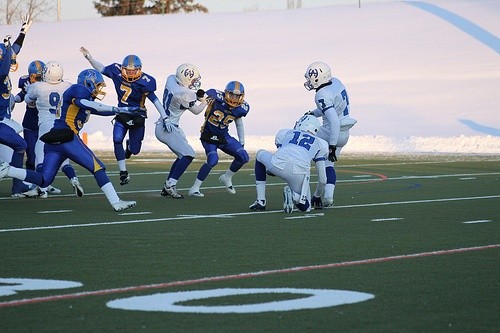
[200,133,228,145]
[38,128,74,145]
[111,114,145,130]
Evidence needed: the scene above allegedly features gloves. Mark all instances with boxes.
[22,81,32,92]
[19,13,33,34]
[163,117,178,132]
[329,145,338,162]
[197,89,204,97]
[4,35,12,47]
[79,45,92,60]
[124,104,148,120]
[304,110,314,115]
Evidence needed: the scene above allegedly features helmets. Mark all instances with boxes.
[175,63,201,90]
[28,60,64,84]
[294,115,323,135]
[121,55,142,82]
[0,42,19,72]
[224,81,245,107]
[77,69,107,101]
[304,61,332,91]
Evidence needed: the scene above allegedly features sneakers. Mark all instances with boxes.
[251,186,334,214]
[162,183,183,198]
[11,177,84,199]
[119,170,131,185]
[218,174,237,195]
[123,139,134,160]
[113,198,136,212]
[0,162,11,182]
[188,190,204,197]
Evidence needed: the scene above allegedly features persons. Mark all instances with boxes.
[304,62,357,207]
[155,63,213,197]
[14,60,46,171]
[0,69,137,212]
[249,114,329,213]
[24,61,84,197]
[0,14,39,198]
[80,46,178,185]
[188,81,249,198]
